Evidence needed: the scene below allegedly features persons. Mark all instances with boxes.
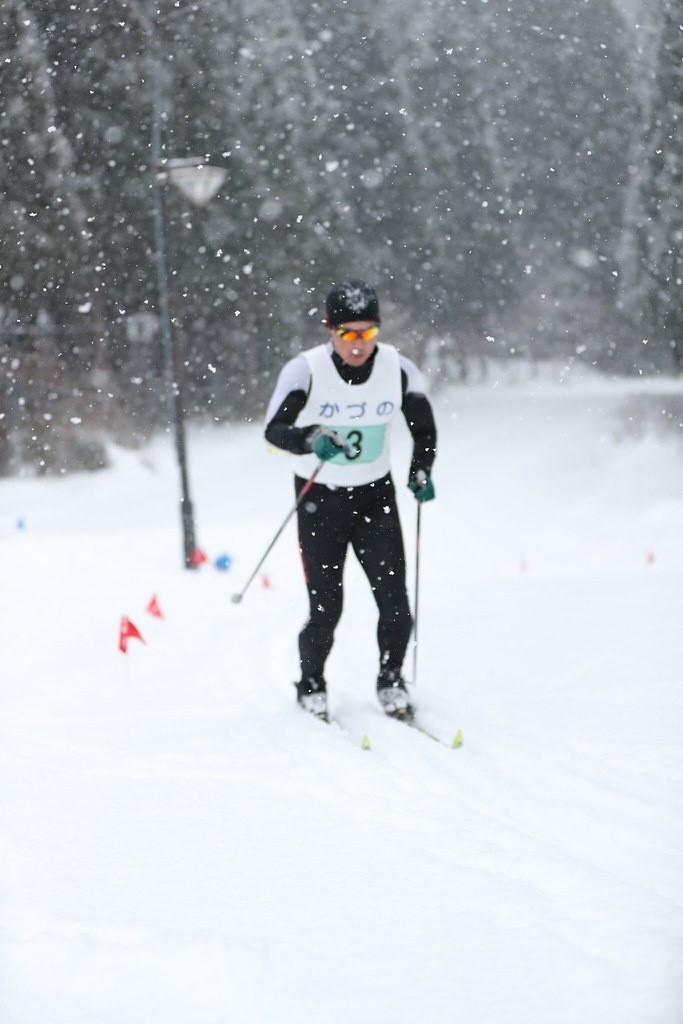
[264,280,437,722]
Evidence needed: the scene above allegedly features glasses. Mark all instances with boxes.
[329,324,380,342]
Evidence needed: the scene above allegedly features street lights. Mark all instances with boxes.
[148,154,235,572]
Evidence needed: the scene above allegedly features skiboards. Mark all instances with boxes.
[291,677,464,755]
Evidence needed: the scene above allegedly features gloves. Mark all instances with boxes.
[310,427,349,460]
[408,472,435,502]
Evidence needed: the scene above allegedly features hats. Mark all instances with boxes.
[326,280,381,328]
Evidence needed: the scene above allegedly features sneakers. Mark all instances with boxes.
[297,671,328,719]
[377,672,416,720]
